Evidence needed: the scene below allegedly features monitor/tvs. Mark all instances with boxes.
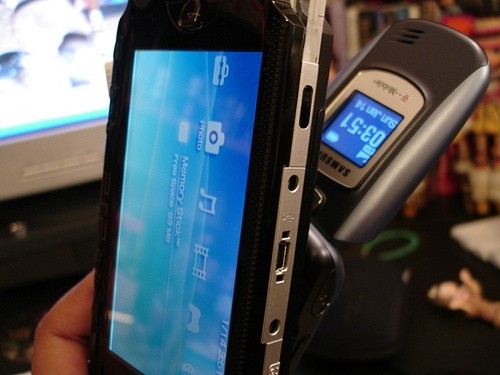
[1,0,130,290]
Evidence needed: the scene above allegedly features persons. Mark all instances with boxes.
[31,267,96,375]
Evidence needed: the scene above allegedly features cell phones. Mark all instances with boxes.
[86,0,336,375]
[278,18,500,375]
